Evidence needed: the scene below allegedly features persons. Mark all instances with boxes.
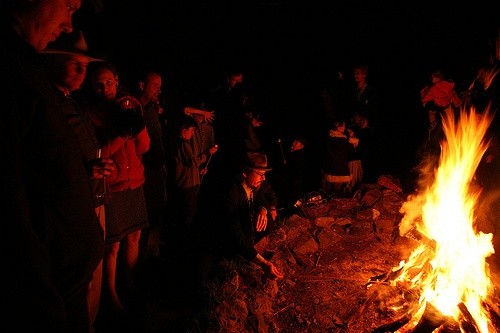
[419,38,500,157]
[339,62,380,157]
[138,69,362,294]
[0,0,149,333]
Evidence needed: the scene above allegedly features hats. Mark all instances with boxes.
[38,28,106,62]
[237,151,272,172]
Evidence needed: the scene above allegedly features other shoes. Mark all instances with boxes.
[108,300,133,317]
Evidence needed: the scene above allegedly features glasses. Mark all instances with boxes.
[250,170,268,179]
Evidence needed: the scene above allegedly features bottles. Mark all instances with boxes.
[92,148,106,199]
